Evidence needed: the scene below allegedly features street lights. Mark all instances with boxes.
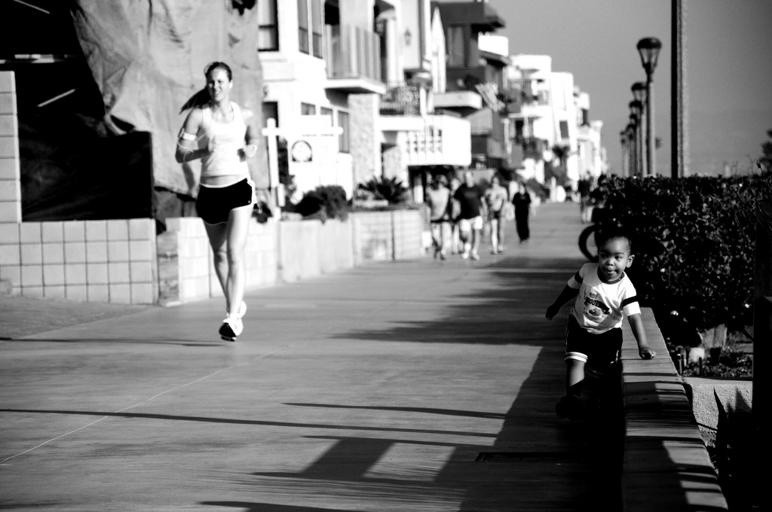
[637,36,661,178]
[620,80,647,178]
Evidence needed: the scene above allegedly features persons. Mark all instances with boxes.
[423,169,533,262]
[543,232,656,439]
[175,61,263,342]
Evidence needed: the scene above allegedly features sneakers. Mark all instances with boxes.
[433,245,503,260]
[219,299,247,343]
